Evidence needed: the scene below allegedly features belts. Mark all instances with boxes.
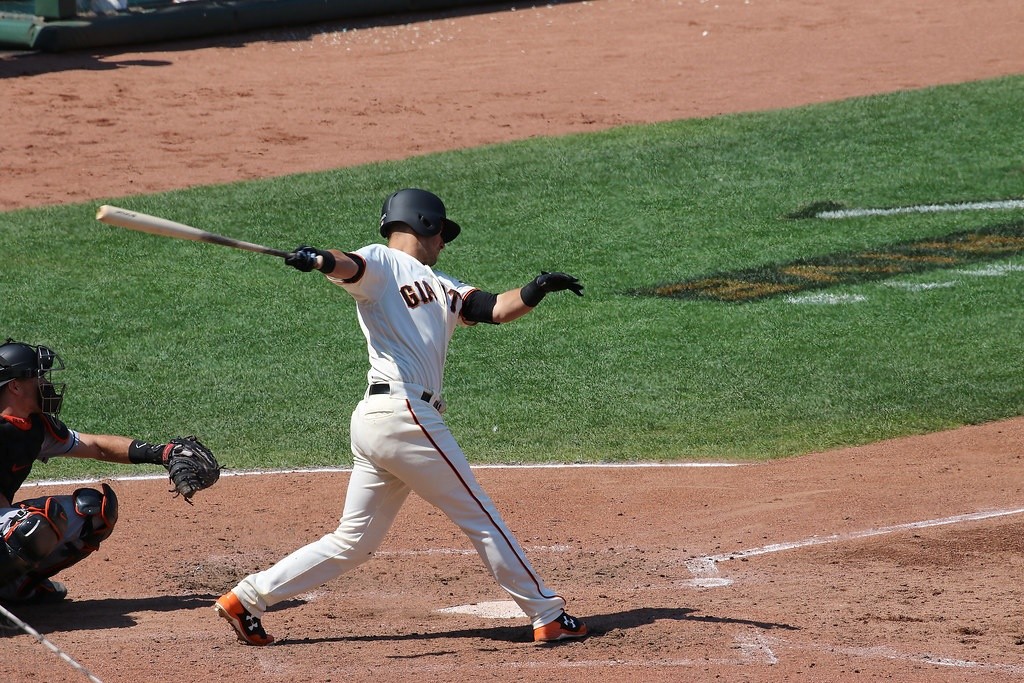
[369,384,442,411]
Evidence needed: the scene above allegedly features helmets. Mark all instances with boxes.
[0,337,37,386]
[379,188,461,243]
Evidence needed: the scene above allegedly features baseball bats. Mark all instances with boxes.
[95,204,323,270]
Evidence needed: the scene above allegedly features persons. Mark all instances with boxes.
[0,339,220,633]
[215,187,587,646]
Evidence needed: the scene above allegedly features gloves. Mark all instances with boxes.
[520,270,584,308]
[285,245,336,275]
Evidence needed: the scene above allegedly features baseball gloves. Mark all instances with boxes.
[161,434,221,499]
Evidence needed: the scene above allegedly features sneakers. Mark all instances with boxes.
[214,591,274,646]
[15,578,68,605]
[534,610,587,642]
